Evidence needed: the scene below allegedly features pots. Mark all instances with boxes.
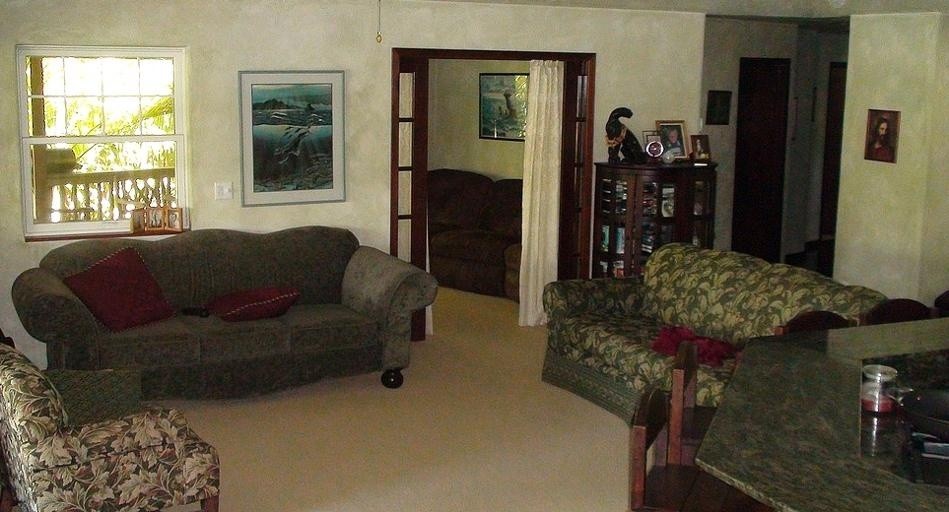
[884,383,949,437]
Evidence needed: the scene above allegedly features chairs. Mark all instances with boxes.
[626,290,949,511]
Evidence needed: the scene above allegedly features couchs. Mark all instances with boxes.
[540,241,890,430]
[10,224,439,402]
[426,165,524,305]
[0,342,220,512]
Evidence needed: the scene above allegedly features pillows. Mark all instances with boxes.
[205,282,303,325]
[60,245,180,334]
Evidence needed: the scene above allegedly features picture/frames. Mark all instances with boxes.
[477,70,530,142]
[238,68,347,208]
[654,116,691,161]
[863,108,902,166]
[128,206,187,234]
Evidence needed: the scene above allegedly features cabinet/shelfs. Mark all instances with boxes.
[591,161,718,280]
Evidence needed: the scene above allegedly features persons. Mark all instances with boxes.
[866,116,894,159]
[695,137,705,152]
[665,127,682,155]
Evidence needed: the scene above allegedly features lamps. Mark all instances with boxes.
[376,1,383,45]
